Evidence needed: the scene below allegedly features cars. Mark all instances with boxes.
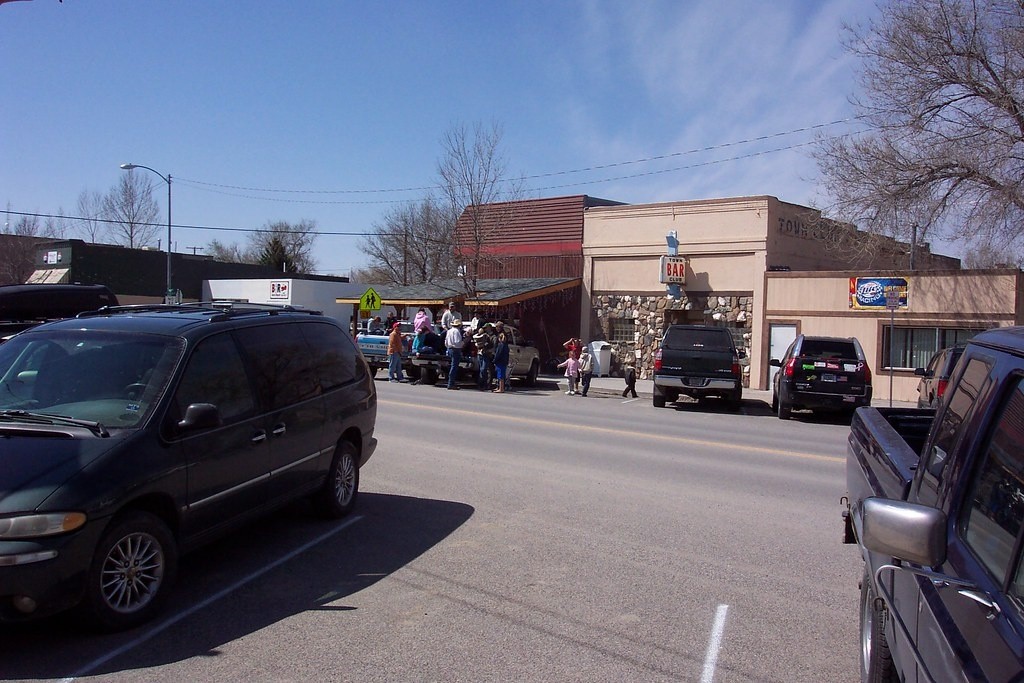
[0,283,121,341]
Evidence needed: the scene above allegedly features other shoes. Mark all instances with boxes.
[389,379,398,383]
[565,391,575,395]
[399,378,409,383]
[582,393,587,397]
[448,386,460,390]
[492,388,504,393]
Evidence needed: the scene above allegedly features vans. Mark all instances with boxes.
[913,342,971,415]
[651,324,747,412]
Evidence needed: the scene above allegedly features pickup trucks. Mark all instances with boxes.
[356,320,449,383]
[836,324,1023,683]
[411,322,542,389]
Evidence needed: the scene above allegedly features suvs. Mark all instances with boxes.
[0,295,381,626]
[769,333,874,421]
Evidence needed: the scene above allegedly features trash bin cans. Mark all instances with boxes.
[587,340,611,377]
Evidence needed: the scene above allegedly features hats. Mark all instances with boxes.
[582,347,588,353]
[473,309,483,314]
[495,321,505,327]
[449,302,455,307]
[393,323,401,328]
[482,323,492,328]
[450,319,461,326]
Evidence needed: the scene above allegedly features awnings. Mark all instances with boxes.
[24,268,70,284]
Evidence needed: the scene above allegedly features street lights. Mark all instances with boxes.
[119,162,174,297]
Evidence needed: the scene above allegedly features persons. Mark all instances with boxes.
[386,322,410,383]
[440,302,510,393]
[557,338,594,397]
[367,316,383,335]
[383,311,397,335]
[412,308,441,356]
[622,362,639,398]
